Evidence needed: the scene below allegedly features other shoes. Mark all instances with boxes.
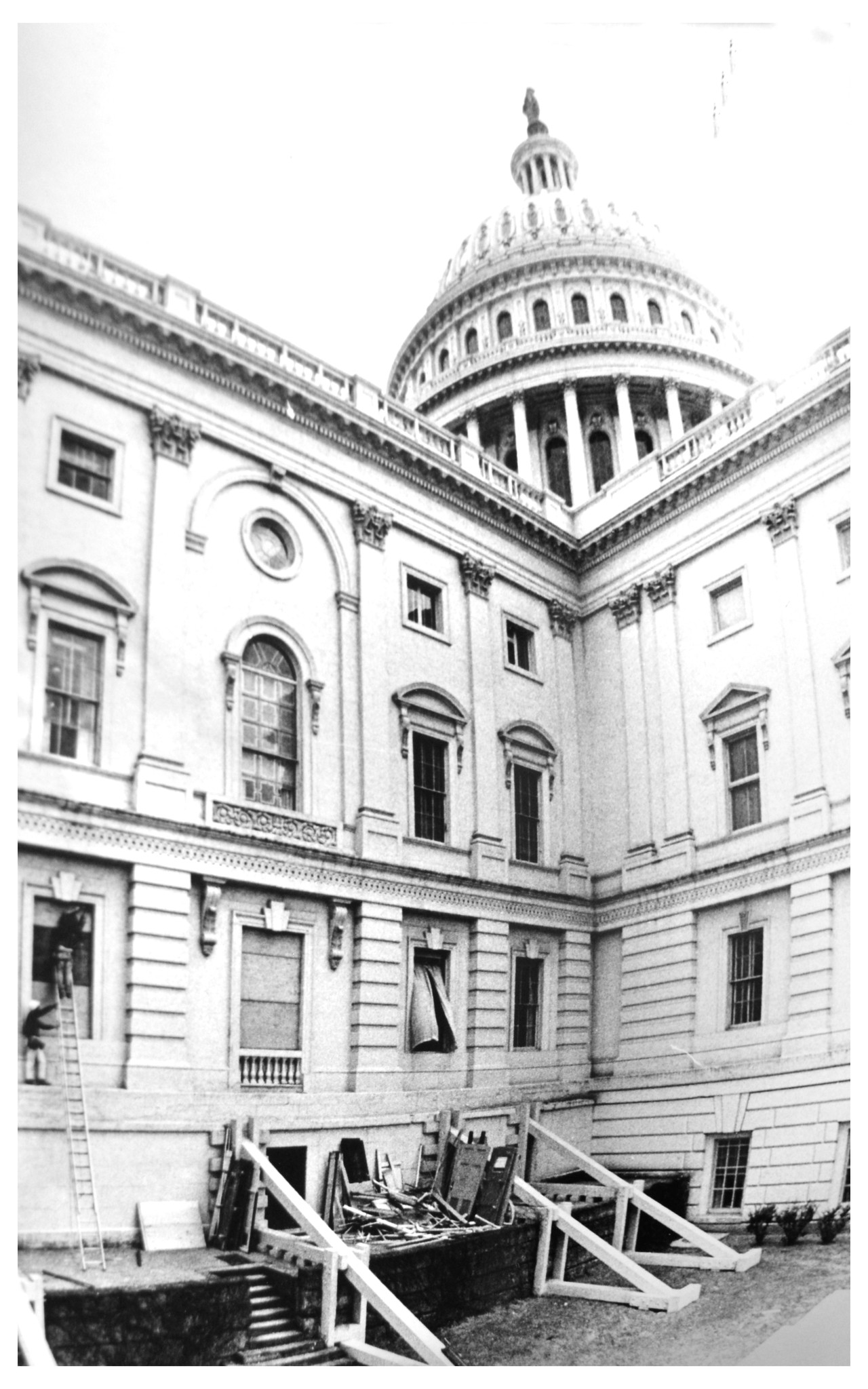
[26,1079,33,1084]
[40,1081,51,1086]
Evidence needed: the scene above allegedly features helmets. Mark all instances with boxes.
[29,1000,41,1010]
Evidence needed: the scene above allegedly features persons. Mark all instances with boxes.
[22,999,60,1085]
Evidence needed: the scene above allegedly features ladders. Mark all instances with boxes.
[54,958,110,1274]
